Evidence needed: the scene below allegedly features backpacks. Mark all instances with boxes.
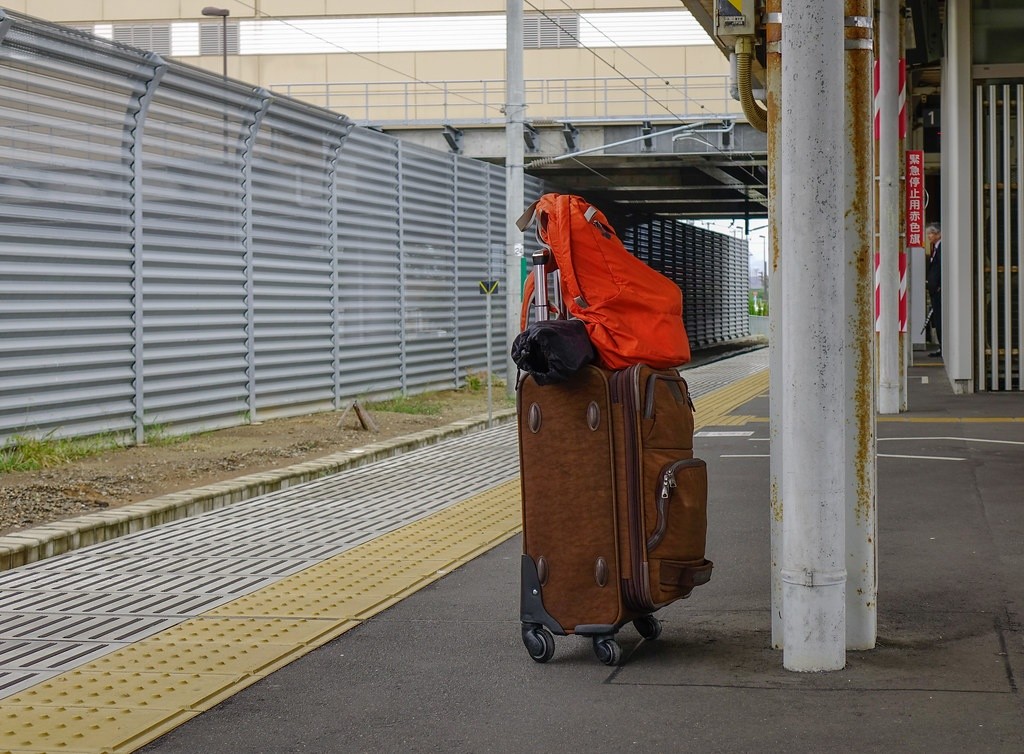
[515,194,693,369]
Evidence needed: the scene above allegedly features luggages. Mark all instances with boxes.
[517,250,714,665]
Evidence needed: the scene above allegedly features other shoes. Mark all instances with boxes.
[927,348,940,357]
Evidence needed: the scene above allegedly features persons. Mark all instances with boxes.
[926,225,941,357]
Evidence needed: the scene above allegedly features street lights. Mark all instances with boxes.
[759,235,767,301]
[736,225,743,240]
[701,222,715,229]
